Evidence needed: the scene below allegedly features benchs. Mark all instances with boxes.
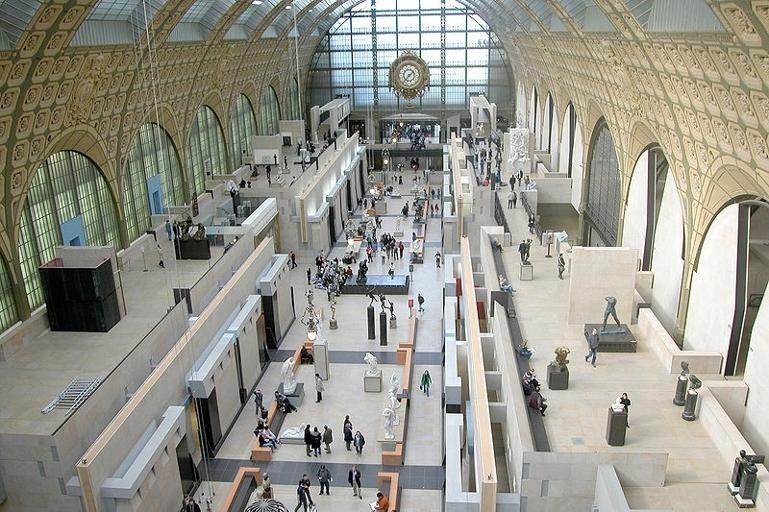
[492,244,552,451]
[220,398,287,512]
[376,471,399,512]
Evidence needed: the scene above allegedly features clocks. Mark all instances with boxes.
[387,47,431,102]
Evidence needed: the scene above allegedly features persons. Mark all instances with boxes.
[287,251,297,269]
[182,372,389,512]
[420,369,432,397]
[584,327,599,368]
[226,129,337,197]
[383,375,399,437]
[513,341,548,417]
[393,157,420,172]
[156,216,205,269]
[307,166,441,312]
[300,346,314,365]
[600,296,622,333]
[284,361,296,387]
[301,286,394,330]
[364,352,377,373]
[499,276,516,297]
[483,170,565,279]
[619,392,631,429]
[223,237,239,254]
[466,122,503,166]
